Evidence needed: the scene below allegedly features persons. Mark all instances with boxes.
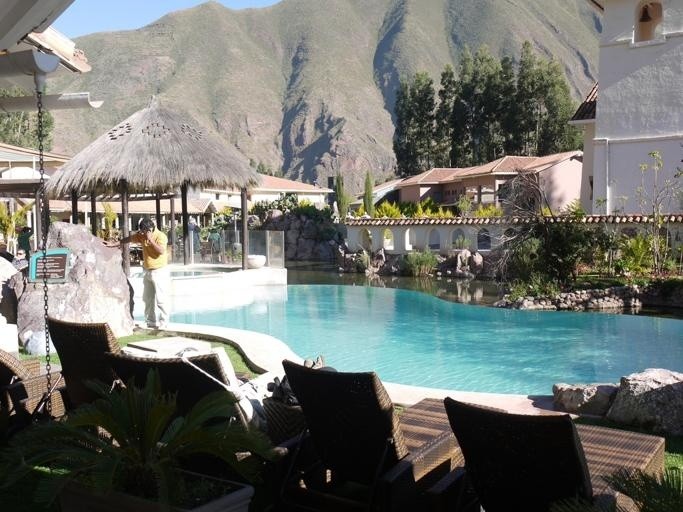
[102,217,170,338]
[187,216,201,255]
[16,226,34,259]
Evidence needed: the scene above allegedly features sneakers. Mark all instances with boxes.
[138,323,169,330]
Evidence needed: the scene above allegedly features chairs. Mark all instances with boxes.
[47,317,124,426]
[258,358,509,512]
[1,346,65,428]
[422,396,666,511]
[102,346,344,503]
